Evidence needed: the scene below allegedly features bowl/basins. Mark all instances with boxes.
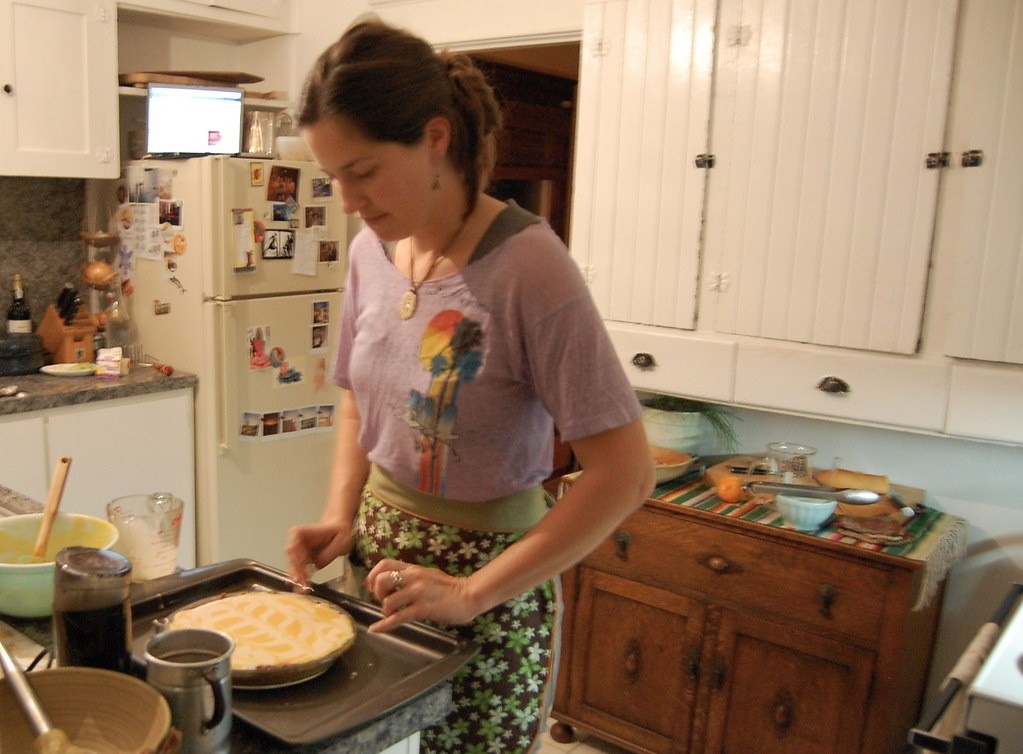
[0,511,120,619]
[654,454,702,486]
[276,135,313,162]
[773,493,837,533]
[0,666,181,754]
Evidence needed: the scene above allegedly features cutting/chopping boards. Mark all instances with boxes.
[704,455,925,537]
[747,480,881,506]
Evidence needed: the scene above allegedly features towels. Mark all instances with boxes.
[922,624,999,754]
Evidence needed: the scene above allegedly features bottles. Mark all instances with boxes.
[5,274,32,336]
[53,545,135,678]
[106,273,129,354]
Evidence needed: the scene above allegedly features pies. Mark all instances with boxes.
[165,592,354,671]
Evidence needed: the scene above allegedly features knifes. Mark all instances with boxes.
[57,282,82,326]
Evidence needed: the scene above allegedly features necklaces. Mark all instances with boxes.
[400,216,467,321]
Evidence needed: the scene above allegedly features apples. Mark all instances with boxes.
[718,479,743,502]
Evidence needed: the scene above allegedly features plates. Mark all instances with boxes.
[157,590,358,691]
[39,363,96,377]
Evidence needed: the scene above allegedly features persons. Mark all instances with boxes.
[286,20,655,753]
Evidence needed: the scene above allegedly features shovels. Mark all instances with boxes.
[16,455,73,565]
[886,489,914,518]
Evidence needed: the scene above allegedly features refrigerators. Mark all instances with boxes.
[90,156,366,584]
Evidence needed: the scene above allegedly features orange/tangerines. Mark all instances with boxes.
[84,261,114,291]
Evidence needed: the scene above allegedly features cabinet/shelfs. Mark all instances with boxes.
[0,0,303,180]
[548,455,973,754]
[555,0,1023,432]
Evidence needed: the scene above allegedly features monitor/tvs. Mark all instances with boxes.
[146,84,245,156]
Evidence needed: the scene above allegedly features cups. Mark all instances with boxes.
[242,109,293,160]
[141,627,236,754]
[748,441,817,485]
[107,491,185,581]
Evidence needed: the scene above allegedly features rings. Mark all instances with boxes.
[392,570,402,589]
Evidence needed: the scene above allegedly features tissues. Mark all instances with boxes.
[95,345,129,379]
